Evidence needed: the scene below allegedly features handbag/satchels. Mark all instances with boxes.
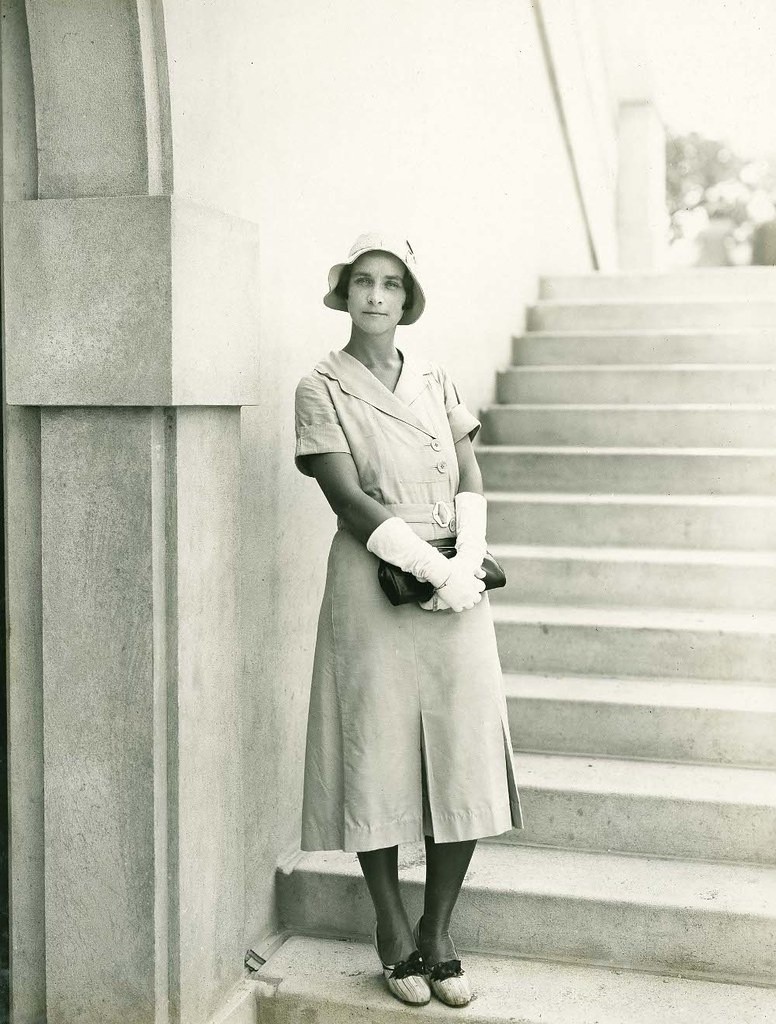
[378,538,506,606]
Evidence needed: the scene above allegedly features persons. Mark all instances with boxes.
[295,230,524,1006]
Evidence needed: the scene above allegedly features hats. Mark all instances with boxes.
[322,232,425,325]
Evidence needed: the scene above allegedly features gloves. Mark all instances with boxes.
[366,516,487,613]
[418,492,487,612]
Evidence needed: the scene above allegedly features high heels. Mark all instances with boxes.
[372,921,432,1006]
[412,914,473,1007]
